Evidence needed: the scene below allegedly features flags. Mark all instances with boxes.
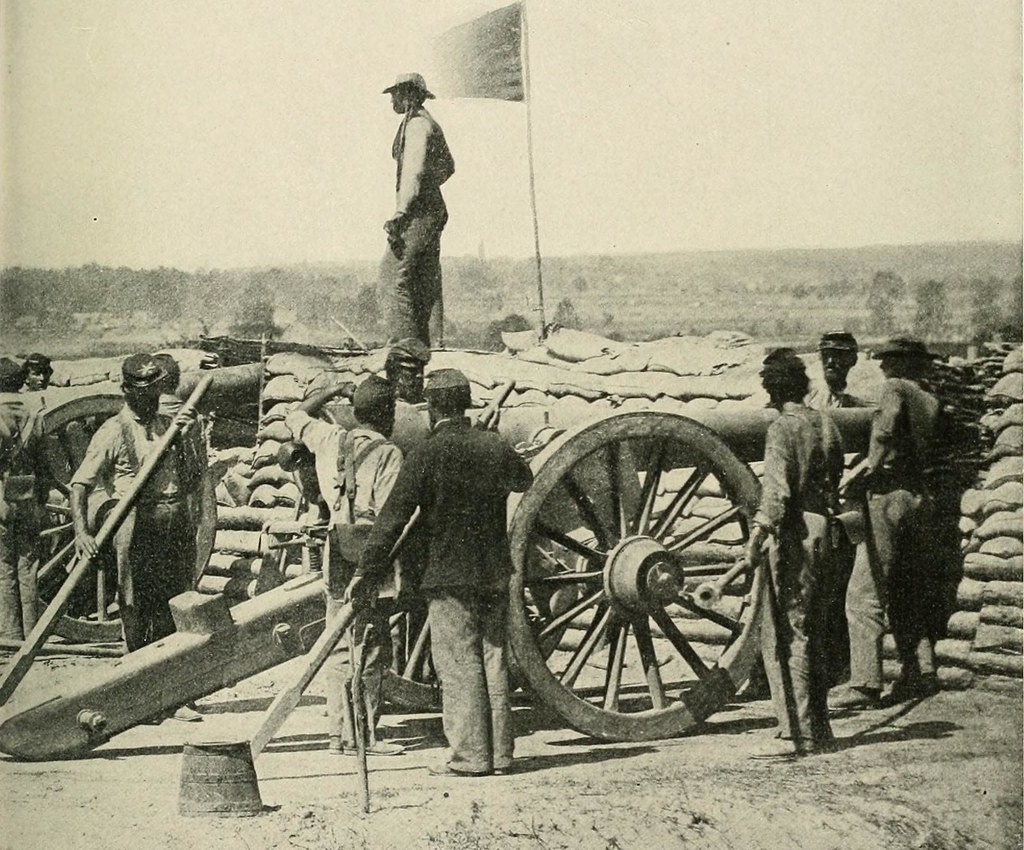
[422,2,531,103]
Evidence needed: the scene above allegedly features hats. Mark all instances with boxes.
[818,331,860,351]
[19,352,53,373]
[154,354,181,377]
[123,354,171,388]
[382,72,437,100]
[387,337,442,362]
[872,338,922,360]
[425,368,471,389]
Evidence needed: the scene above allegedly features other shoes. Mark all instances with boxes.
[426,755,513,779]
[919,668,940,699]
[327,734,405,757]
[824,679,884,712]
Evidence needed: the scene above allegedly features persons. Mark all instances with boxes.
[382,73,454,347]
[0,339,534,775]
[734,330,945,760]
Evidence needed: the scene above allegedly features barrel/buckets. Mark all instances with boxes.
[176,740,262,820]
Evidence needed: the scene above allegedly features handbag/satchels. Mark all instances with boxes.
[324,524,398,601]
